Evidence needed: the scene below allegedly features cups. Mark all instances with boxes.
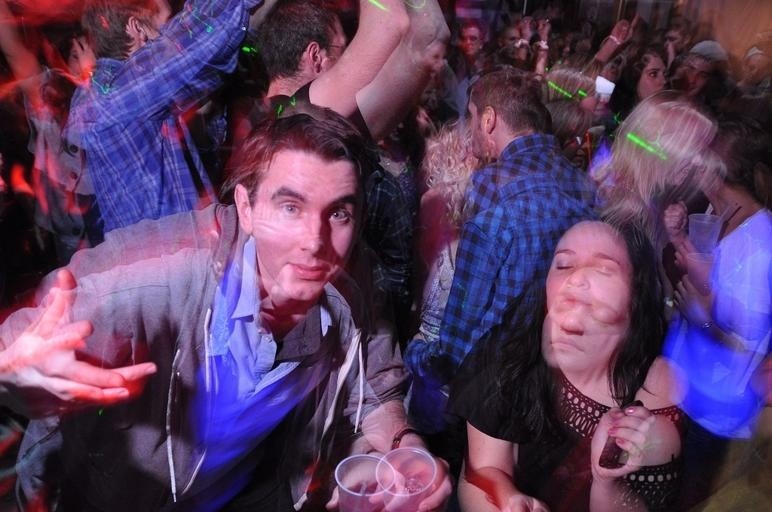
[685,253,713,294]
[688,211,721,252]
[377,446,437,512]
[335,453,396,512]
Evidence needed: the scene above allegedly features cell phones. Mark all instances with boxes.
[599,400,644,468]
